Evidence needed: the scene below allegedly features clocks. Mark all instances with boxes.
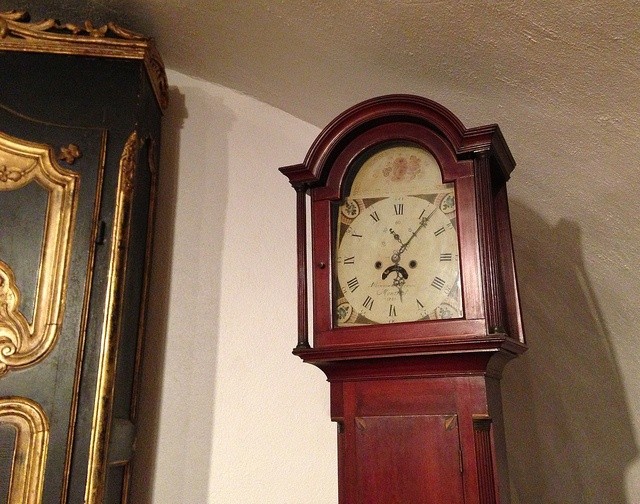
[327,138,467,330]
